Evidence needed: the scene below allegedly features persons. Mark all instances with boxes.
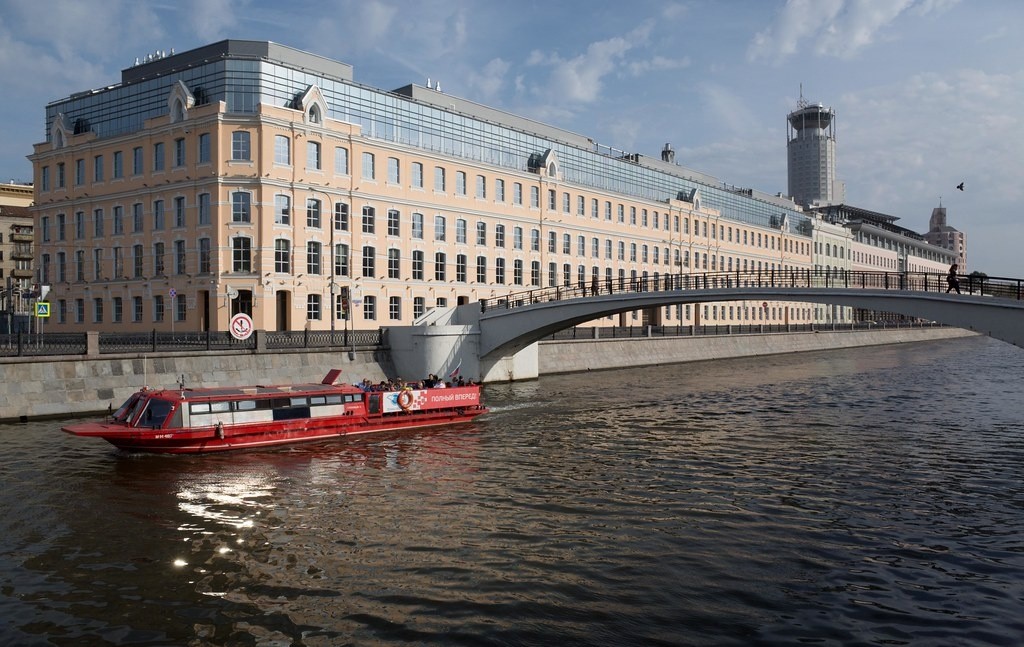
[592,276,598,295]
[944,264,961,294]
[360,374,476,392]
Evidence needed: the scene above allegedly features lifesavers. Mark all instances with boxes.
[397,389,414,408]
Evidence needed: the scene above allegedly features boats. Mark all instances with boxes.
[60,358,490,454]
